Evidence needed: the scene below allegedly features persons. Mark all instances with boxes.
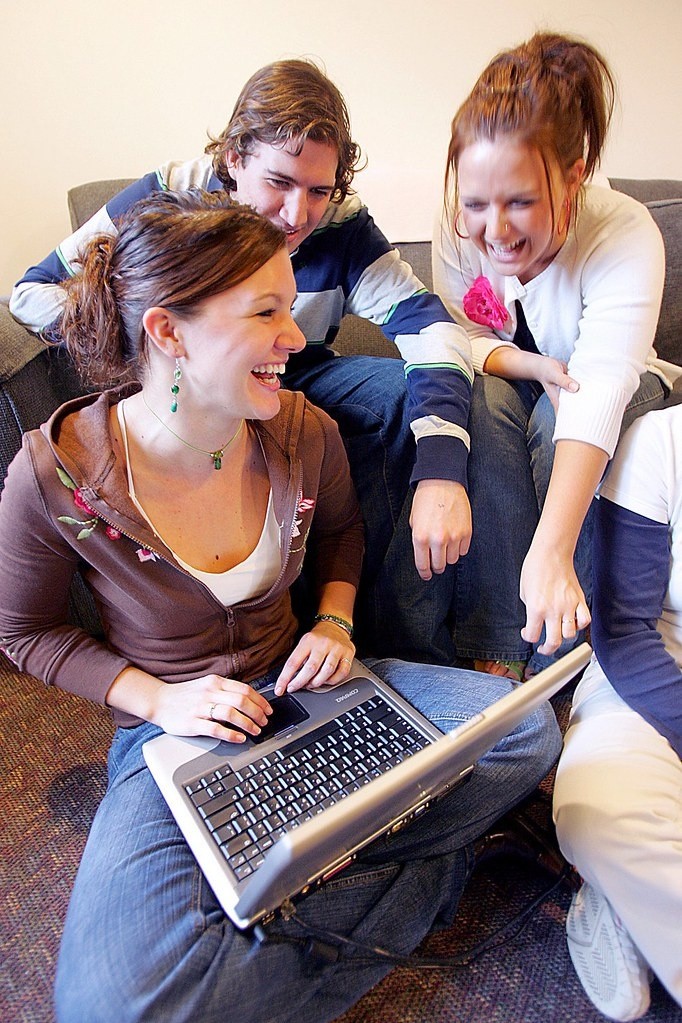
[8,60,476,669]
[432,35,681,680]
[552,404,682,1023]
[1,189,562,1023]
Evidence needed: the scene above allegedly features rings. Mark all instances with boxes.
[340,658,353,667]
[209,703,218,720]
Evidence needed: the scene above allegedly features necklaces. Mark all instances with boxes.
[141,392,243,469]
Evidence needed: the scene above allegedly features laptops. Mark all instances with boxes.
[142,642,593,945]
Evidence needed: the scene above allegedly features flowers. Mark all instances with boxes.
[463,275,510,330]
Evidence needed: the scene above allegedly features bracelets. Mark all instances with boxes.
[314,613,354,639]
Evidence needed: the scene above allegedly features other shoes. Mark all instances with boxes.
[565,883,651,1022]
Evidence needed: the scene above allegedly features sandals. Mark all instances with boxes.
[486,660,526,681]
